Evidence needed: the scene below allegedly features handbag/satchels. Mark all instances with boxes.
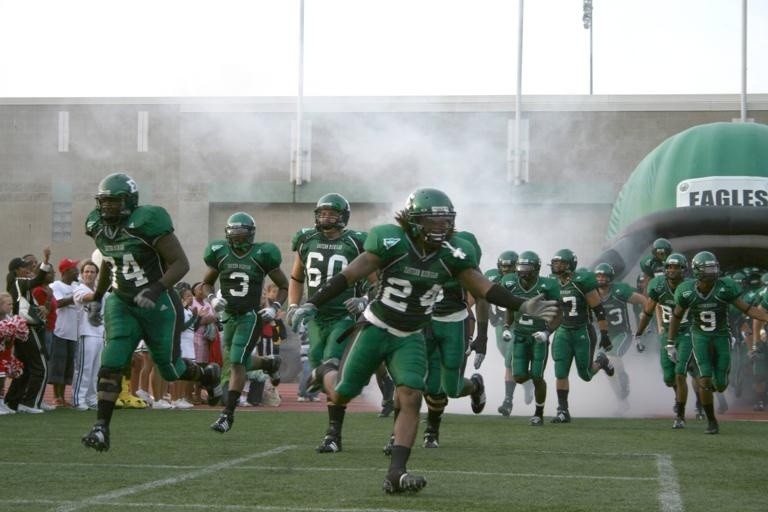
[204,324,216,342]
[17,296,48,328]
[273,325,287,346]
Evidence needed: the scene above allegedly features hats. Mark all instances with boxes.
[59,259,80,272]
[8,258,33,271]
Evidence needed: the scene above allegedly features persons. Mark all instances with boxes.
[201,211,288,432]
[2,246,320,415]
[284,194,373,455]
[292,189,558,494]
[82,173,222,454]
[370,237,767,434]
[381,230,486,457]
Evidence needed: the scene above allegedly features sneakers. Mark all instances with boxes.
[316,432,342,453]
[266,355,281,385]
[498,401,513,414]
[597,353,614,376]
[0,399,98,415]
[82,425,108,451]
[735,385,742,399]
[377,400,394,418]
[673,399,728,434]
[116,389,193,408]
[755,400,763,411]
[210,414,234,434]
[201,362,223,405]
[425,430,439,448]
[307,359,337,393]
[529,411,571,424]
[619,375,629,398]
[238,402,252,407]
[297,396,320,402]
[383,468,426,491]
[471,374,486,414]
[523,381,535,405]
[384,438,393,456]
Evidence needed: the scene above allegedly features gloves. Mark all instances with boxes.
[666,343,680,365]
[133,282,164,308]
[749,344,762,363]
[285,301,320,333]
[635,335,642,351]
[256,303,279,322]
[532,330,549,343]
[760,330,767,341]
[503,324,509,339]
[87,294,106,327]
[465,336,488,369]
[519,294,557,323]
[342,295,369,312]
[208,293,225,313]
[598,331,611,350]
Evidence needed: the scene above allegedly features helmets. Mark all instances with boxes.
[637,272,644,290]
[653,238,688,282]
[405,189,456,248]
[225,212,256,250]
[733,266,762,287]
[94,174,138,226]
[498,249,577,278]
[315,193,351,232]
[595,262,614,287]
[692,251,720,281]
